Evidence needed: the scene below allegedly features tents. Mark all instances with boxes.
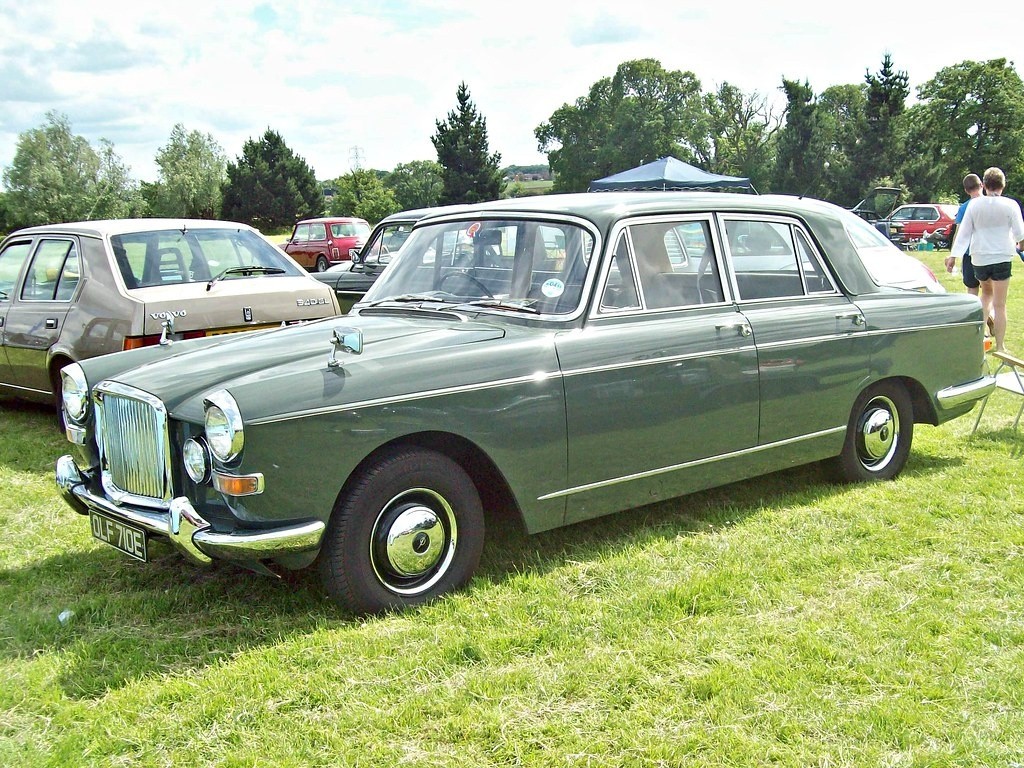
[590,156,750,193]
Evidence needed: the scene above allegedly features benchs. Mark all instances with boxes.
[663,274,821,307]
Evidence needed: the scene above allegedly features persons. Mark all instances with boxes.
[945,174,986,296]
[947,167,1024,354]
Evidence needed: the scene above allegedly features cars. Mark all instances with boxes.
[875,204,964,245]
[57,189,997,620]
[275,204,816,316]
[0,219,342,415]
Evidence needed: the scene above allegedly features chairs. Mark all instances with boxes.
[526,287,716,313]
[972,351,1024,436]
[150,246,189,283]
[936,223,957,252]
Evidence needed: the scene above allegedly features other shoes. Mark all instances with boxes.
[987,315,995,337]
